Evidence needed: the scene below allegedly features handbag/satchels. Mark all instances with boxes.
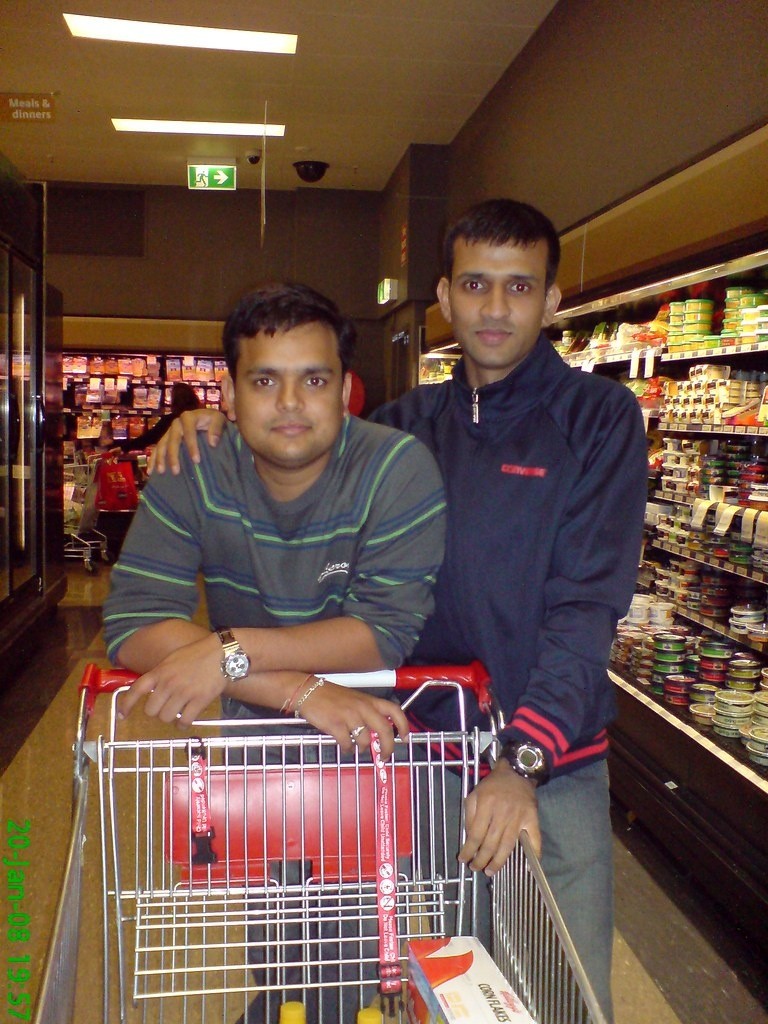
[93,452,138,511]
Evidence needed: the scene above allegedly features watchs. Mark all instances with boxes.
[498,737,551,788]
[215,626,251,683]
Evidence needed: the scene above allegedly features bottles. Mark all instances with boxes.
[357,1008,382,1024]
[280,1001,306,1024]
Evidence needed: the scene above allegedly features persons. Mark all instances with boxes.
[106,383,201,462]
[144,198,647,1023]
[98,277,446,1022]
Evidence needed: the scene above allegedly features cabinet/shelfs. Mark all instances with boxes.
[454,341,767,819]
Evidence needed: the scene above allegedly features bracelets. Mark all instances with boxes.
[278,672,326,719]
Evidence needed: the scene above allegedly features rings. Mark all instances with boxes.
[350,725,365,742]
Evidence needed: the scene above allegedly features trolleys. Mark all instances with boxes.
[61,454,113,575]
[33,665,606,1024]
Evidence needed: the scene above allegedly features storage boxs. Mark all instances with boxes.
[404,935,537,1023]
